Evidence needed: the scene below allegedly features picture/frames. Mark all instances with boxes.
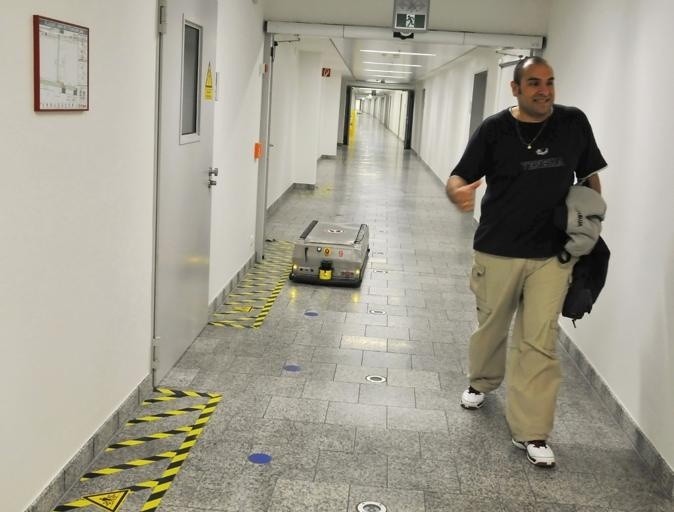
[33,15,90,112]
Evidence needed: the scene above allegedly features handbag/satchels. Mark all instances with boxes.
[558,235,610,320]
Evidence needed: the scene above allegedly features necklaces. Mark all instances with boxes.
[515,108,552,149]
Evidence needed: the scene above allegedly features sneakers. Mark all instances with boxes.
[462,385,486,410]
[511,435,555,467]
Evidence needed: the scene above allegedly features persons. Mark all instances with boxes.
[445,55,609,469]
